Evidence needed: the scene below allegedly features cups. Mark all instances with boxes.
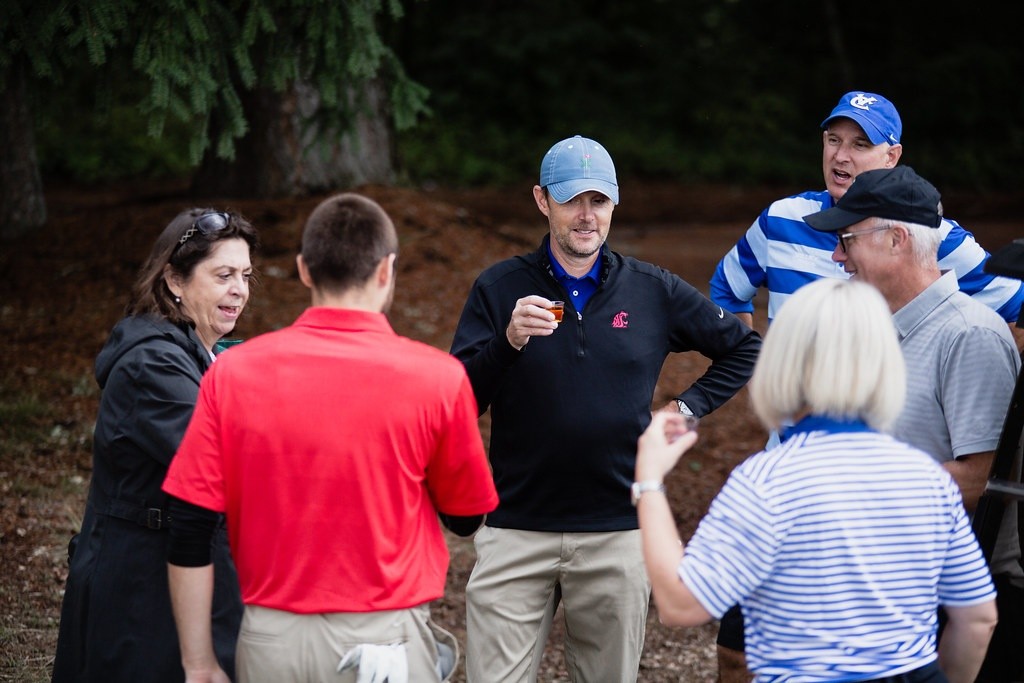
[663,413,700,445]
[545,301,564,322]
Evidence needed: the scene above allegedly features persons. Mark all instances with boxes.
[51,208,259,683]
[635,277,998,683]
[160,193,499,683]
[451,134,762,683]
[710,92,1024,333]
[802,167,1024,682]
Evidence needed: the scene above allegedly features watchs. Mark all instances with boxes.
[677,400,693,417]
[631,482,664,506]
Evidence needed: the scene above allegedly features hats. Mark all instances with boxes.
[820,89,902,147]
[802,161,942,232]
[539,135,618,205]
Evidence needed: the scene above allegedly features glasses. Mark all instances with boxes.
[833,224,910,252]
[170,212,232,258]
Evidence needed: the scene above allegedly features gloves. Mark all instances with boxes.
[336,644,408,683]
